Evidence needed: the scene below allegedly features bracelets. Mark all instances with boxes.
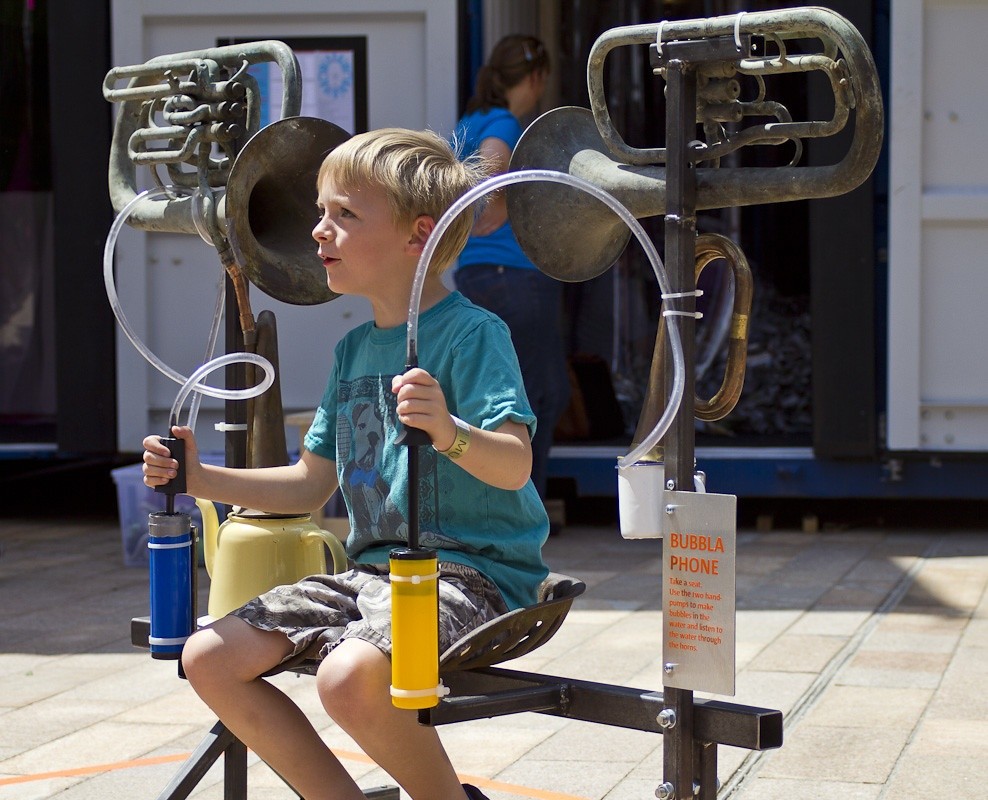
[432,413,470,457]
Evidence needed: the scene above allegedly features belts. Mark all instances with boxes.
[482,265,509,273]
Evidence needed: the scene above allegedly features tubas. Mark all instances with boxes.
[505,6,883,282]
[103,39,353,307]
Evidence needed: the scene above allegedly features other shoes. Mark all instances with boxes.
[462,784,490,800]
[547,505,565,535]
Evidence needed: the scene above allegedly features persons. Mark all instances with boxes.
[444,33,568,538]
[143,130,549,797]
